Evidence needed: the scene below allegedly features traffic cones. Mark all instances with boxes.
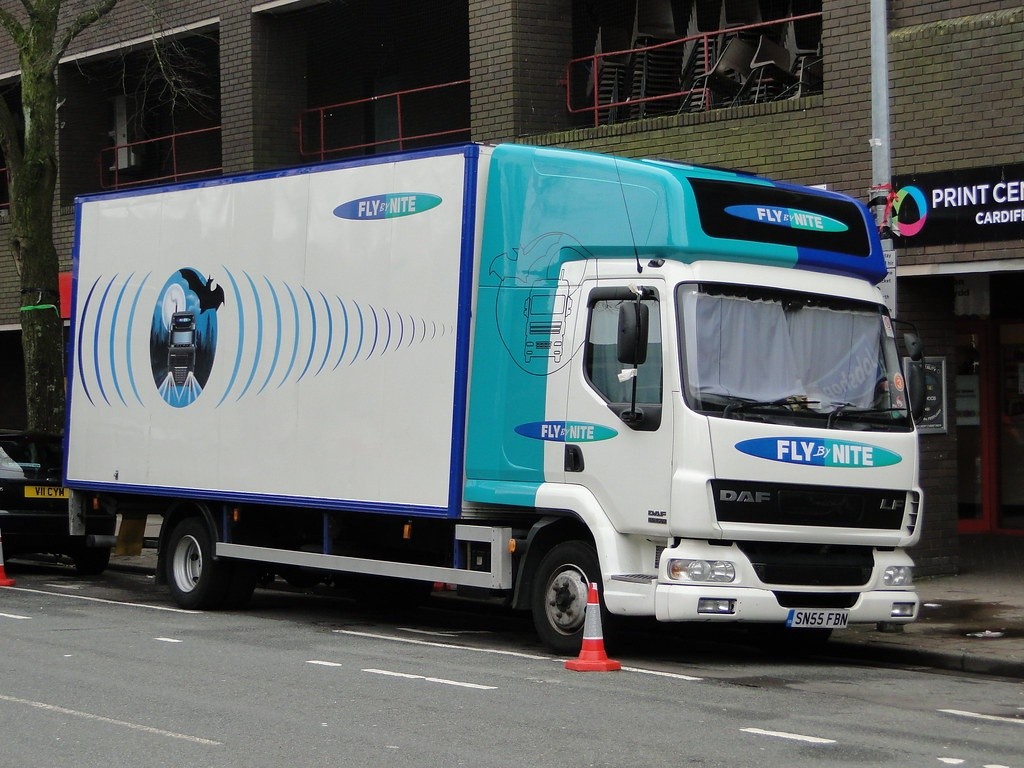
[0,540,16,586]
[565,581,622,671]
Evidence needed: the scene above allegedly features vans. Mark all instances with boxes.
[0,317,117,578]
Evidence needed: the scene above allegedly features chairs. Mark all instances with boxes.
[574,0,823,125]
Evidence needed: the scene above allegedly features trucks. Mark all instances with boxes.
[60,139,928,657]
